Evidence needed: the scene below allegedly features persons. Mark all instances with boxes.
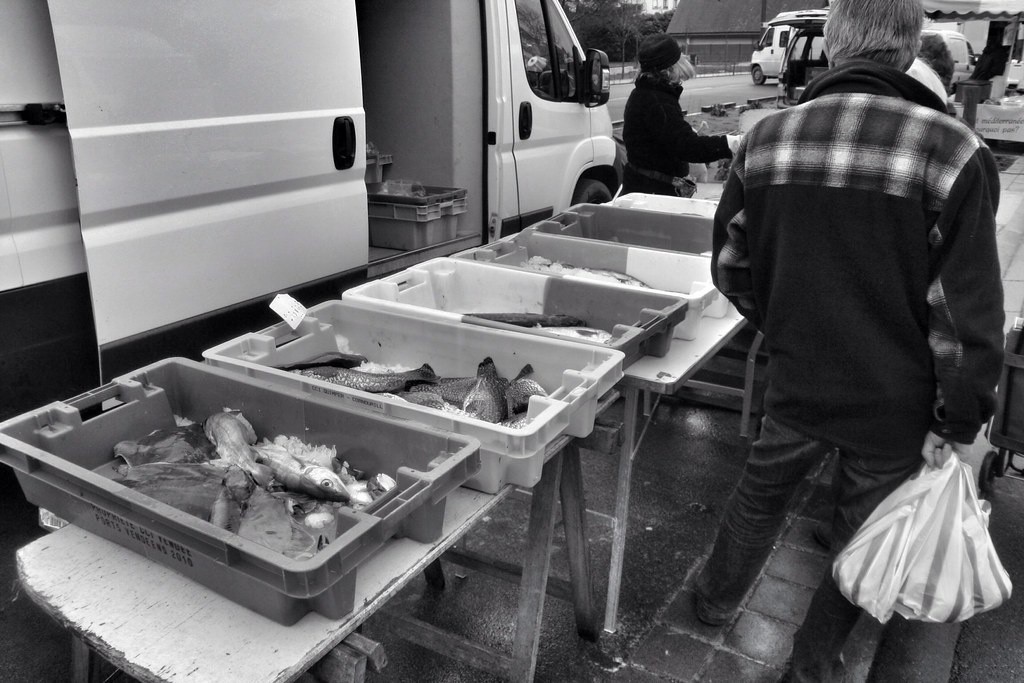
[694,0,1003,683]
[904,33,954,106]
[622,34,742,195]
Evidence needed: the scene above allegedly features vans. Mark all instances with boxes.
[749,10,981,110]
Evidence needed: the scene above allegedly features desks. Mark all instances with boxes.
[15,392,621,683]
[518,298,761,630]
[10,388,627,683]
[949,96,1024,148]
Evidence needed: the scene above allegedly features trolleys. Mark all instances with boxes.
[977,312,1024,492]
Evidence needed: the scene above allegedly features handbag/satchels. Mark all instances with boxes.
[829,451,1015,626]
[629,162,697,198]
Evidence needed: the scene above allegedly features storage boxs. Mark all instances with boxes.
[202,299,628,495]
[0,351,483,627]
[364,153,468,251]
[442,227,720,346]
[521,203,719,257]
[952,80,994,104]
[339,252,696,370]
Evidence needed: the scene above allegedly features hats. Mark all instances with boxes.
[637,34,682,73]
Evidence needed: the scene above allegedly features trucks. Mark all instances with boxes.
[0,0,624,405]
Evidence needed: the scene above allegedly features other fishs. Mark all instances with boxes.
[466,309,589,327]
[109,408,396,559]
[540,261,653,288]
[269,352,551,428]
[533,324,613,344]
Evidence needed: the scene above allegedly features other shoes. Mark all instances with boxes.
[693,561,734,626]
